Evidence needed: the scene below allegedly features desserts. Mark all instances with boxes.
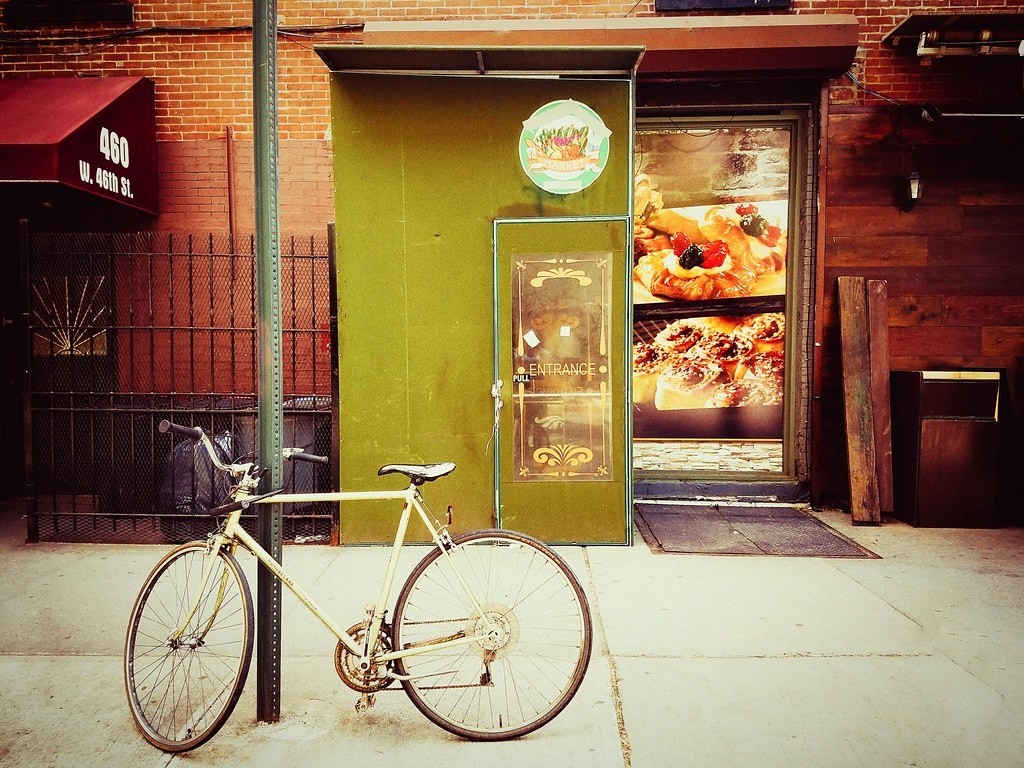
[633,313,784,409]
[636,205,787,300]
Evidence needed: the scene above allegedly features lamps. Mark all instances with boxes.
[902,166,924,212]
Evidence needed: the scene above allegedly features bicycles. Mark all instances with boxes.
[125,416,594,753]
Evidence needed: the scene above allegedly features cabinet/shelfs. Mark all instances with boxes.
[891,369,1006,530]
